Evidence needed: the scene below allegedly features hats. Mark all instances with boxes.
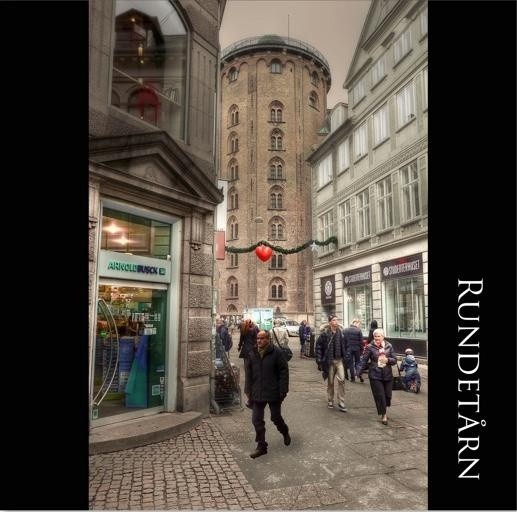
[328,314,336,321]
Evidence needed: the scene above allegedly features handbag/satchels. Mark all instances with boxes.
[318,352,329,371]
[392,376,403,390]
[281,347,293,362]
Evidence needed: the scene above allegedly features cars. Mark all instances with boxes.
[277,319,301,336]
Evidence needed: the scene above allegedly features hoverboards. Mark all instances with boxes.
[398,369,421,393]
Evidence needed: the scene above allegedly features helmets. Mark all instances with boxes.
[405,348,413,355]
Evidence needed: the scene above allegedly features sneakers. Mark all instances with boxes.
[338,402,347,412]
[283,426,290,446]
[359,375,364,382]
[251,442,268,458]
[327,400,333,409]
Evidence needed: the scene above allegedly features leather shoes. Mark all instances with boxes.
[382,413,387,425]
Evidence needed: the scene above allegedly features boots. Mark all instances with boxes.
[300,352,306,358]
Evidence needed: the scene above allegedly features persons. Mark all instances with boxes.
[214,312,419,459]
